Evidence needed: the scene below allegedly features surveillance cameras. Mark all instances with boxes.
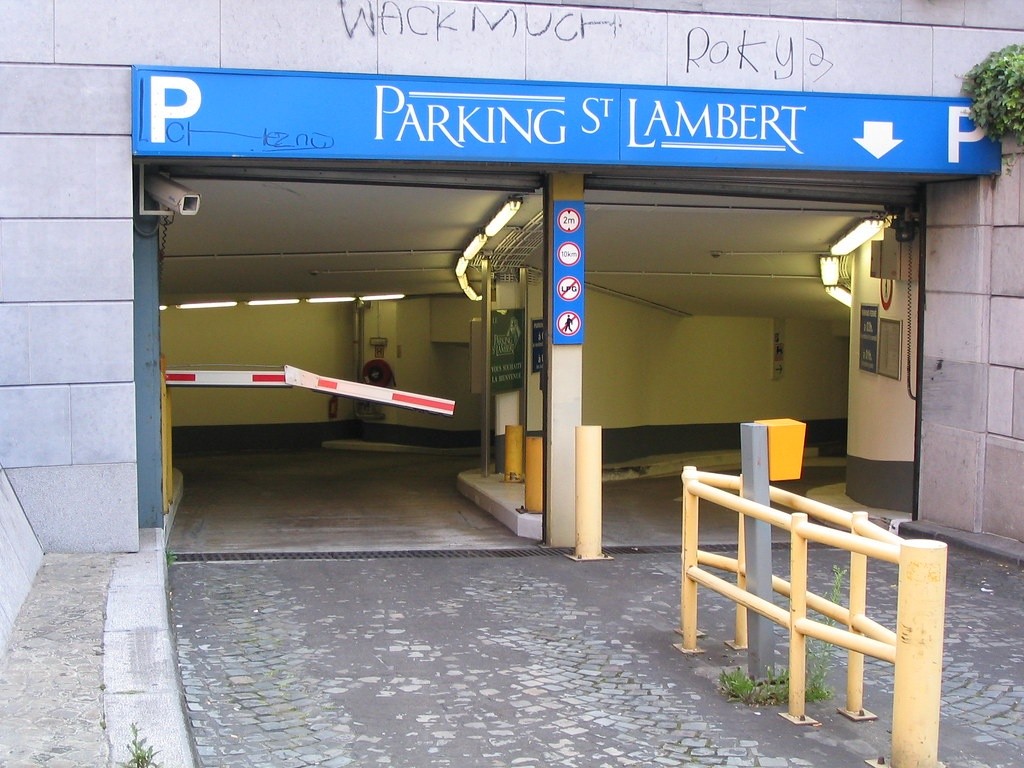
[144,172,202,215]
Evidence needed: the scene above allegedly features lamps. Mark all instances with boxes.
[820,255,840,286]
[829,218,886,256]
[823,285,851,307]
[484,197,523,237]
[463,231,489,260]
